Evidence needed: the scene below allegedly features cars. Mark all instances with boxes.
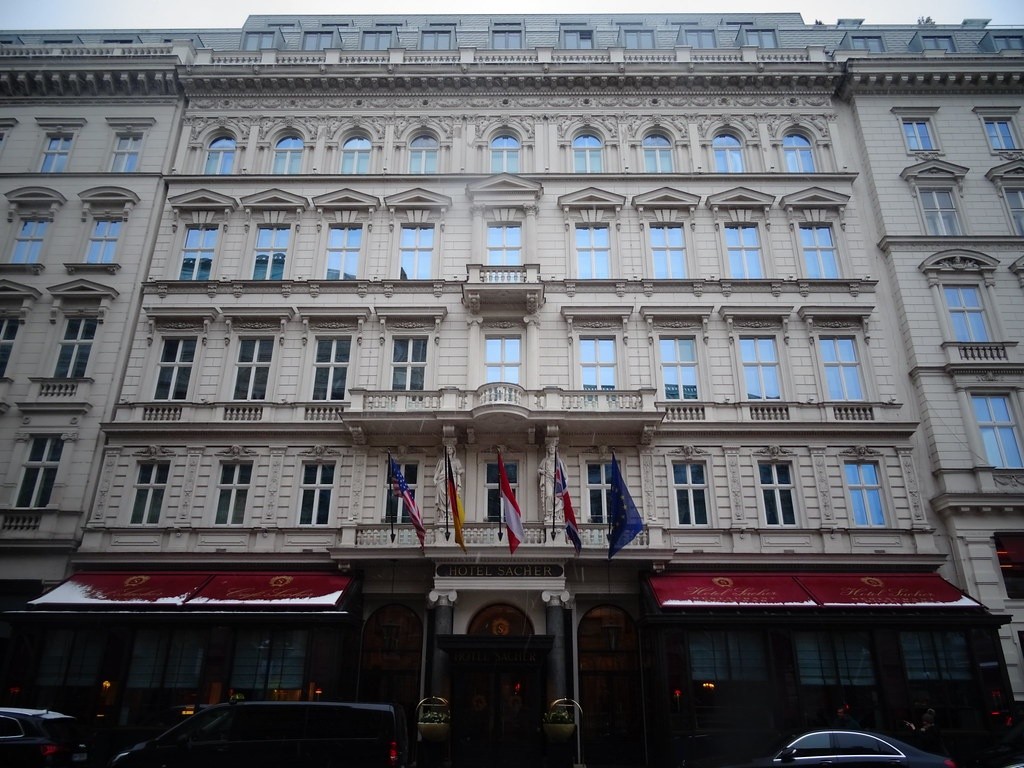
[969,713,1024,768]
[718,727,956,768]
[0,704,90,768]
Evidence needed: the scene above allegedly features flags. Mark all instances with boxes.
[385,447,428,554]
[554,452,588,556]
[442,454,471,556]
[497,453,527,554]
[607,451,644,561]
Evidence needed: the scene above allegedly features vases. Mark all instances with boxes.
[416,722,449,739]
[545,722,577,738]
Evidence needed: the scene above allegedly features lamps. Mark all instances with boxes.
[602,624,621,650]
[381,623,400,653]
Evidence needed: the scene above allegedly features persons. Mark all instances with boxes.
[909,708,943,754]
[433,445,465,522]
[537,444,567,521]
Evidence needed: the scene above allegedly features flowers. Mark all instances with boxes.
[420,711,449,723]
[541,709,575,725]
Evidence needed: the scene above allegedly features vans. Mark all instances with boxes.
[104,695,409,768]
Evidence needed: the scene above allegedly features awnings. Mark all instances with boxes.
[0,570,364,623]
[646,569,997,621]
